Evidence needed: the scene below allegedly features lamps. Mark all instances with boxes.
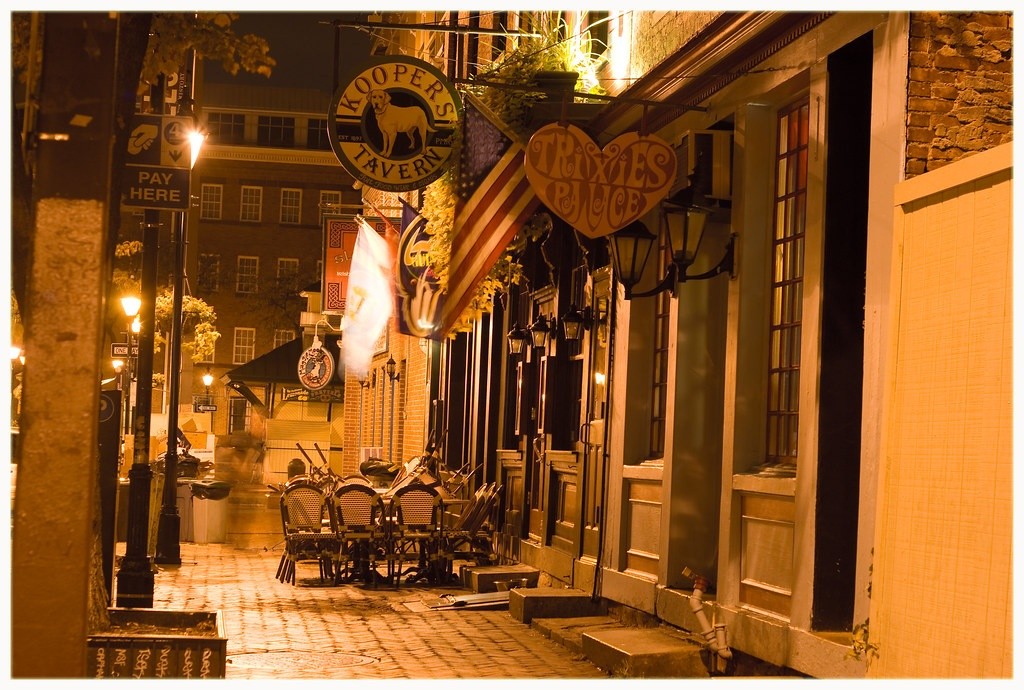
[529,312,558,350]
[561,305,591,341]
[607,217,676,300]
[507,323,532,354]
[655,164,737,282]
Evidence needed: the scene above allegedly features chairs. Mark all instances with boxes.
[276,474,508,584]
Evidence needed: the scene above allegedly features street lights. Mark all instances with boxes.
[152,112,211,566]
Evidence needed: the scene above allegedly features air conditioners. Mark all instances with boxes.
[662,127,734,213]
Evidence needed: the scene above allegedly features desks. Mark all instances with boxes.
[383,498,470,507]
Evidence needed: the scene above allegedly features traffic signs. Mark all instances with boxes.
[120,294,140,434]
